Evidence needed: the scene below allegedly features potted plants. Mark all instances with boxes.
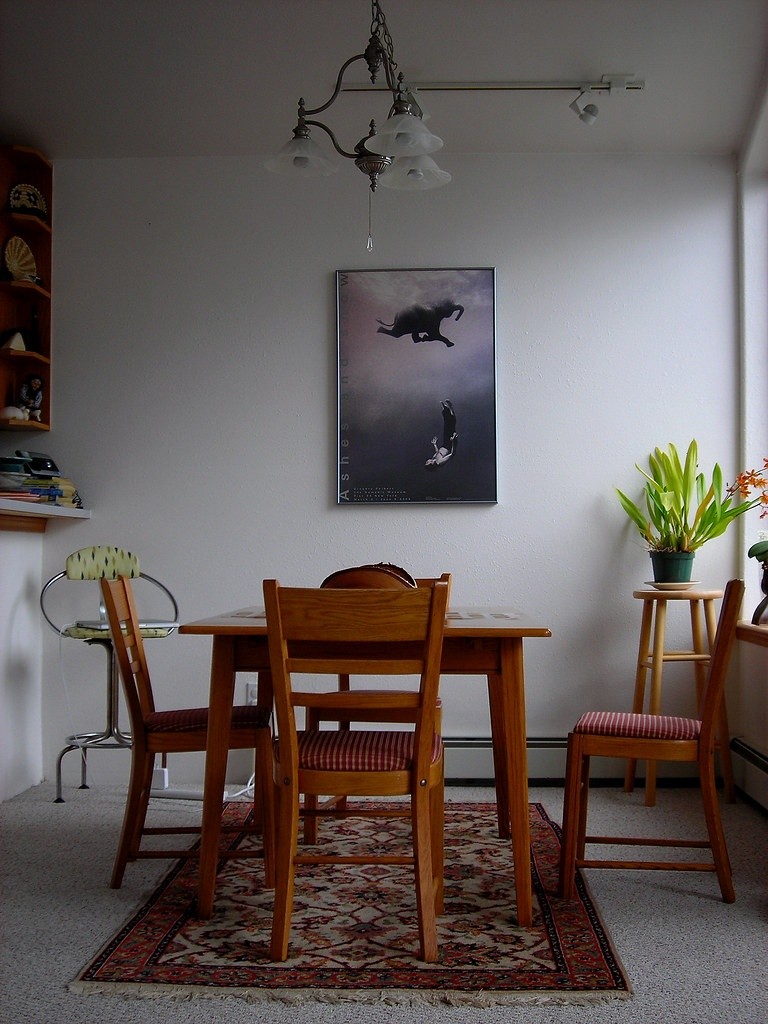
[618,439,768,584]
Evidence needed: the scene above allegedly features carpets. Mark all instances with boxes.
[67,801,632,1009]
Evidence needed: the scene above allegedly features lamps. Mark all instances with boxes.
[567,84,600,125]
[265,0,452,254]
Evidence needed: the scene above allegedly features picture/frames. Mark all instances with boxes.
[337,267,499,505]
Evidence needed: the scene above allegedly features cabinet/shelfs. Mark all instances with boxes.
[0,145,53,431]
[624,591,734,808]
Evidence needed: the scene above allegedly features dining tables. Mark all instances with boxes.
[178,607,553,927]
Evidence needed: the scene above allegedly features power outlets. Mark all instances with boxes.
[245,683,258,706]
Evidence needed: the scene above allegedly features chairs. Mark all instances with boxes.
[261,579,451,962]
[305,574,444,845]
[40,545,182,804]
[98,573,276,889]
[558,577,745,903]
